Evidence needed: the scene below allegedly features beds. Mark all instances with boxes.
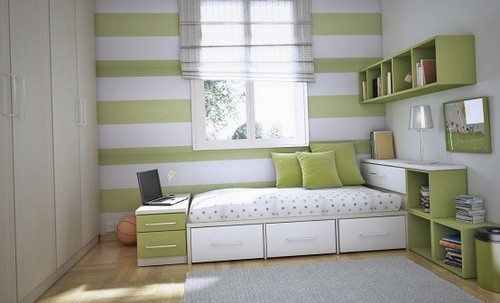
[186,187,408,263]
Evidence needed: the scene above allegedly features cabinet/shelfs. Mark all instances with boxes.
[1,0,101,303]
[135,193,190,267]
[358,35,477,104]
[360,156,498,280]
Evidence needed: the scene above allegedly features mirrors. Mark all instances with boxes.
[443,96,492,155]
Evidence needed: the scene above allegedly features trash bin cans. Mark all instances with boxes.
[474,226,500,293]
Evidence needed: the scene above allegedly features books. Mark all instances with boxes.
[361,57,436,100]
[418,186,486,268]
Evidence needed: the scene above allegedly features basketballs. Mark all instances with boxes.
[115,215,137,246]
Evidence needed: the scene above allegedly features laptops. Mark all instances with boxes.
[137,169,188,205]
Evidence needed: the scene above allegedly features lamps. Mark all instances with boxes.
[409,106,440,166]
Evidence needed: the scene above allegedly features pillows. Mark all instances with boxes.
[270,142,365,189]
